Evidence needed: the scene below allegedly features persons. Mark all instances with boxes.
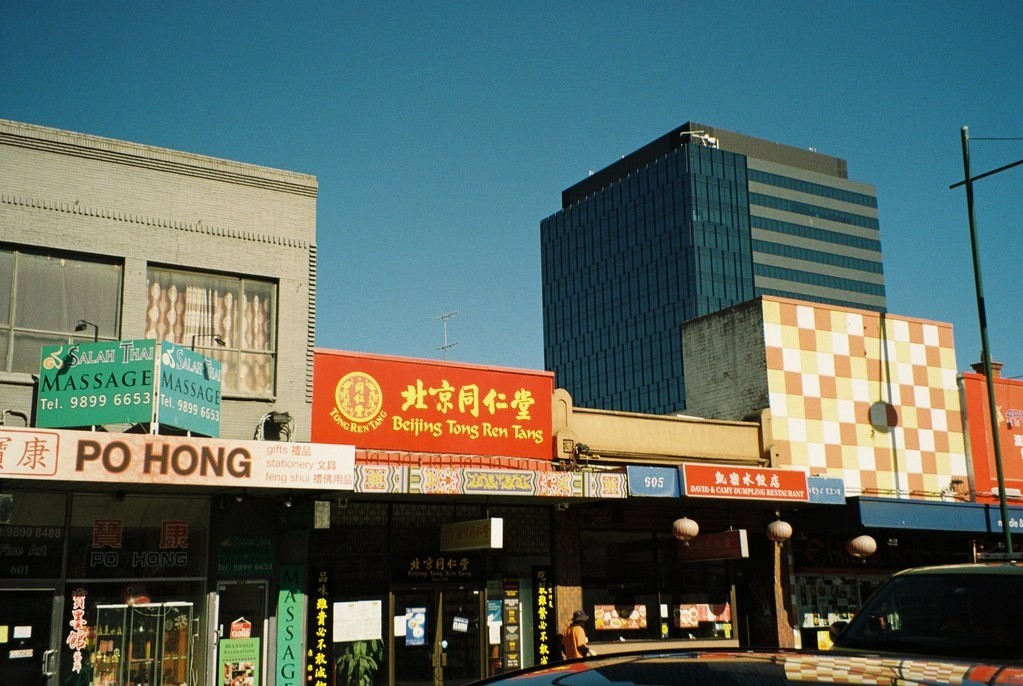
[562,610,594,660]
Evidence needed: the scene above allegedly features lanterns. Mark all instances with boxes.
[767,520,792,546]
[847,533,877,563]
[672,517,699,546]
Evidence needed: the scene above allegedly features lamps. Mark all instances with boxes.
[75,319,100,342]
[191,334,225,352]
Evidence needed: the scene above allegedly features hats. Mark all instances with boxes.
[571,611,589,621]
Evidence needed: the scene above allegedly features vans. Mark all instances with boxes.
[828,562,1023,667]
